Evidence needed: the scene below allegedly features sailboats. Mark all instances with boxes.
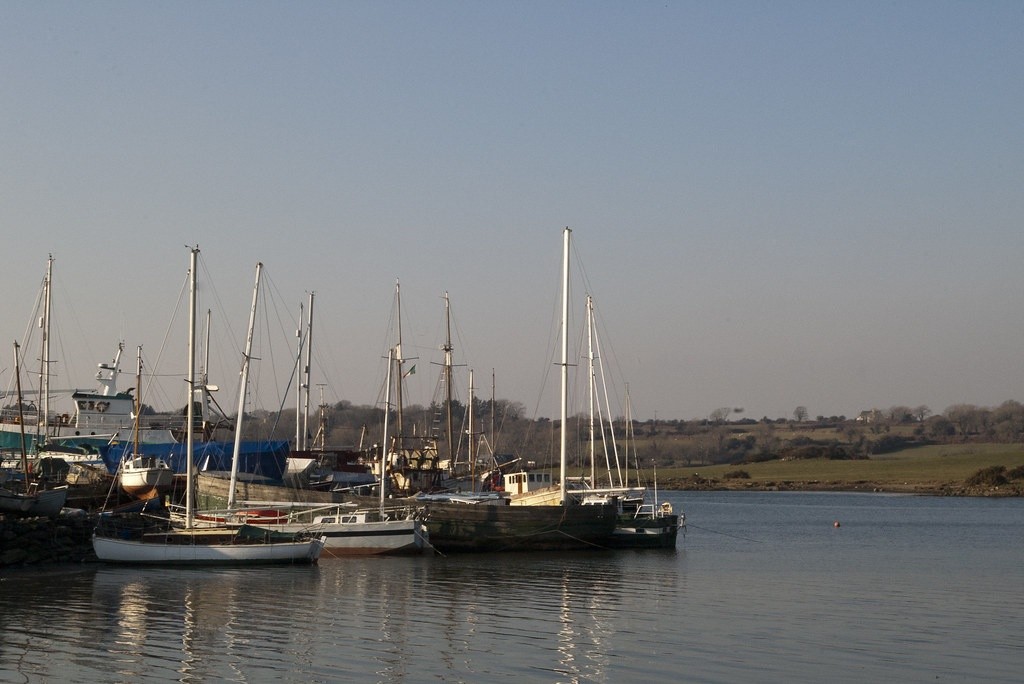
[0,224,678,565]
